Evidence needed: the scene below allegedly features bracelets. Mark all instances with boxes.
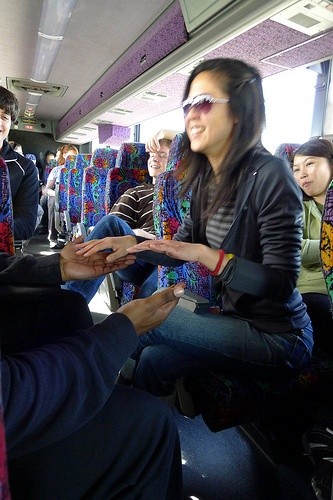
[207,249,224,275]
[225,253,234,260]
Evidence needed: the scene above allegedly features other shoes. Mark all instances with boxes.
[49,240,60,249]
[38,228,47,235]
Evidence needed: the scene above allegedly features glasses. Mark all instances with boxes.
[181,94,231,119]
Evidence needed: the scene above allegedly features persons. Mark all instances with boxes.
[75,56,314,400]
[0,85,333,500]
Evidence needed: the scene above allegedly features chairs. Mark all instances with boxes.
[0,142,299,313]
